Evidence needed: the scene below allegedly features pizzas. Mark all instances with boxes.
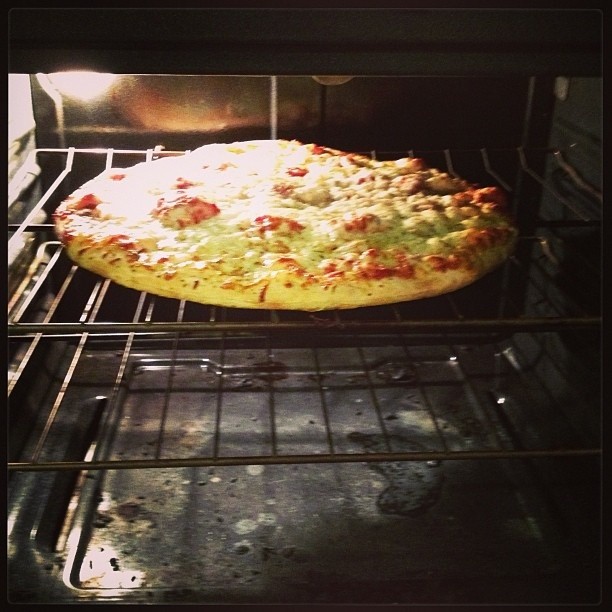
[55,139,520,312]
